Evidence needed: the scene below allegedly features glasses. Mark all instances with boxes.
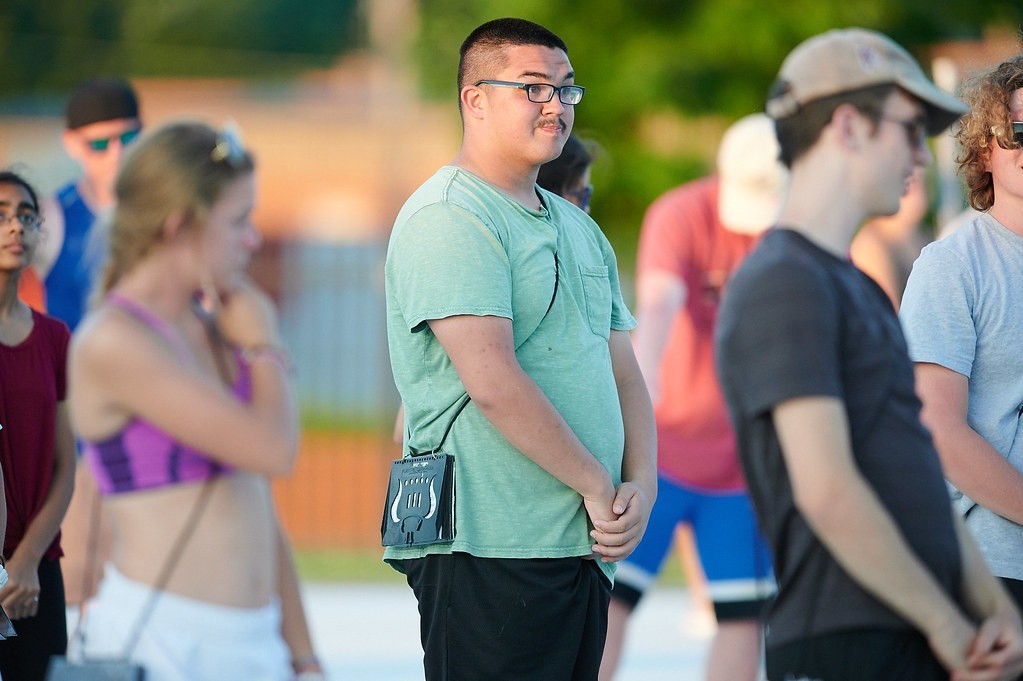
[564,184,594,214]
[0,210,47,227]
[86,130,140,150]
[209,116,249,171]
[878,114,930,150]
[991,122,1023,149]
[472,78,585,108]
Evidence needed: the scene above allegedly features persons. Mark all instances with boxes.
[385,17,1021,681]
[0,76,330,681]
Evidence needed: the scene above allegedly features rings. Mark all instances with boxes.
[33,598,39,603]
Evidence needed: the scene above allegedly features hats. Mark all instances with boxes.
[716,112,792,235]
[67,76,137,130]
[766,24,972,137]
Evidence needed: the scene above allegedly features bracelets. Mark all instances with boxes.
[241,344,295,377]
[293,655,322,672]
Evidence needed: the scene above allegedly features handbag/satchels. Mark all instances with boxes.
[381,448,457,549]
[45,655,146,681]
